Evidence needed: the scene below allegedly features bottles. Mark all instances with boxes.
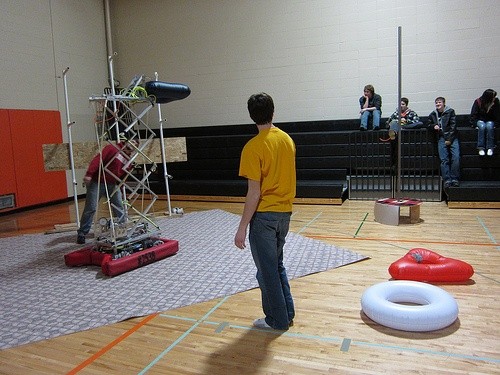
[154,72,159,81]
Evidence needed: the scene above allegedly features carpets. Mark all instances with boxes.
[0,208,370,349]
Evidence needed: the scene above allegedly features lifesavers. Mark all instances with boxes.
[127,81,190,104]
[361,280,459,331]
[389,248,474,282]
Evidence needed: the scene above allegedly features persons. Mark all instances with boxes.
[469,89,500,155]
[77,138,139,245]
[359,85,382,131]
[385,98,424,139]
[235,93,296,330]
[427,97,461,188]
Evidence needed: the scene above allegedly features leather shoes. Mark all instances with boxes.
[77,234,85,244]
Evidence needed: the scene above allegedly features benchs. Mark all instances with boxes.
[122,114,500,205]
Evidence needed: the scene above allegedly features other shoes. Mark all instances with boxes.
[163,207,184,216]
[445,183,450,188]
[374,126,378,129]
[487,149,493,156]
[479,149,485,155]
[360,127,367,130]
[389,130,395,140]
[453,182,459,187]
